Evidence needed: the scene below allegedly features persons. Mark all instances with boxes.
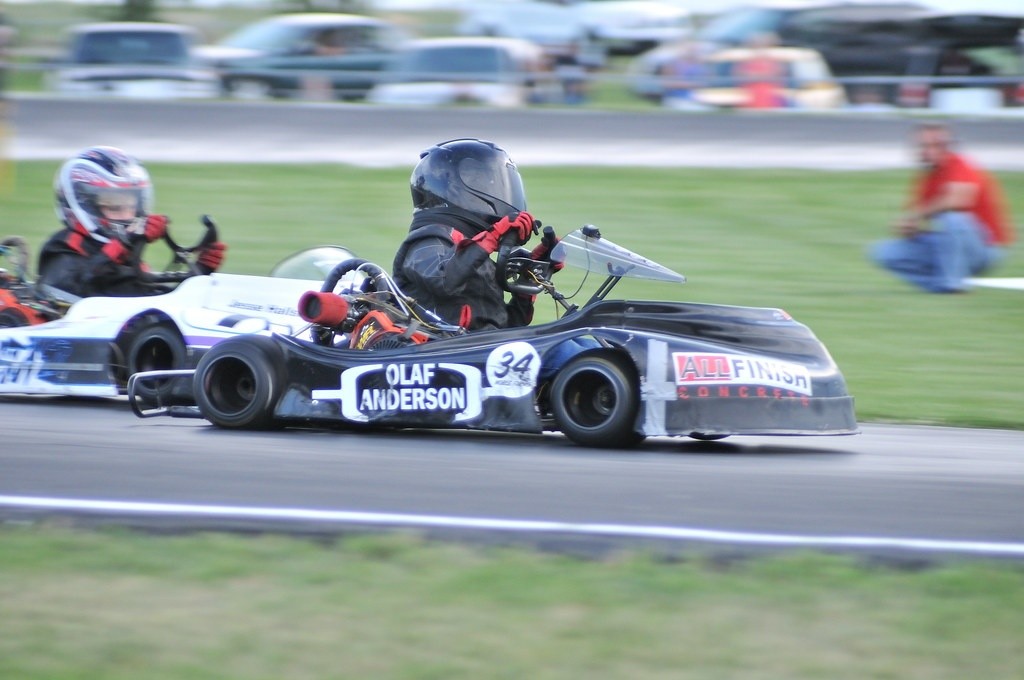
[519,57,597,106]
[391,136,563,334]
[36,144,230,294]
[294,30,374,104]
[871,123,1008,293]
[660,33,796,108]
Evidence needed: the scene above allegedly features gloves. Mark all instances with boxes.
[511,236,567,302]
[471,210,539,254]
[197,242,226,269]
[101,214,166,264]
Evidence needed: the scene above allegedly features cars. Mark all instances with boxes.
[49,21,225,102]
[367,0,1023,114]
[199,16,404,105]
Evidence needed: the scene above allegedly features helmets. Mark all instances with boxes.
[53,146,154,244]
[410,137,531,252]
